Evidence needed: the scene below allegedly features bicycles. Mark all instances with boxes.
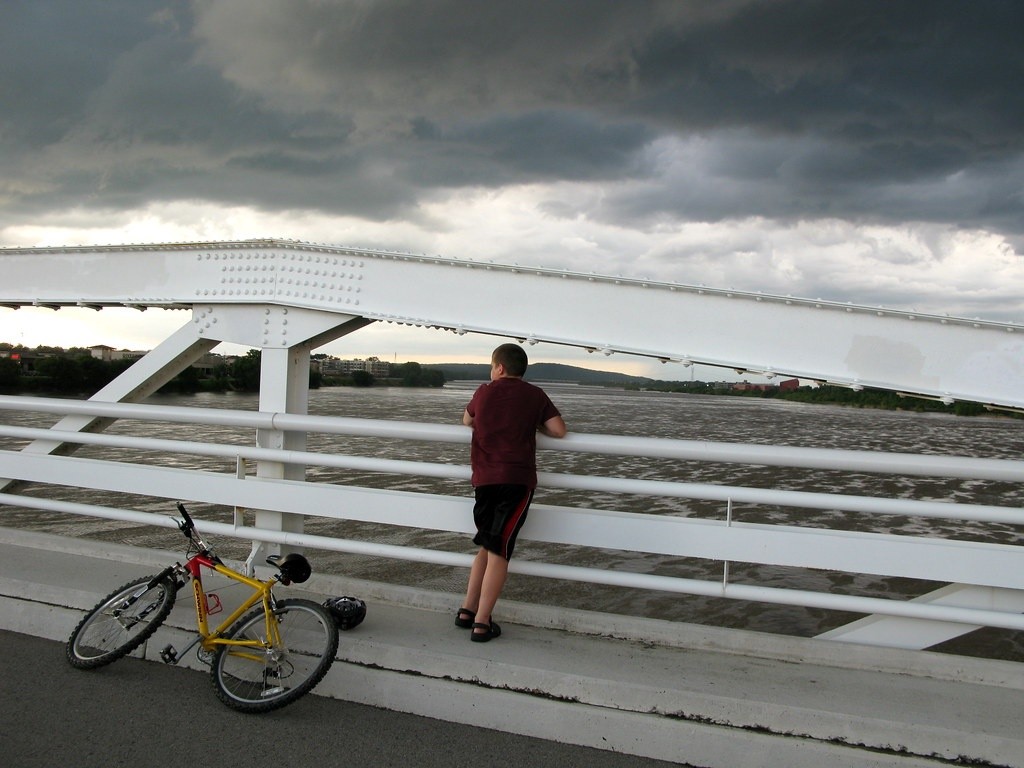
[65,500,351,713]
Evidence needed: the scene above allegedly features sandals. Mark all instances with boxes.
[455,607,476,629]
[471,615,501,643]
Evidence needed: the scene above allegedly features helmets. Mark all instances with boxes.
[318,595,367,630]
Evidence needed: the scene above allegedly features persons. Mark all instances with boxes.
[454,343,567,643]
